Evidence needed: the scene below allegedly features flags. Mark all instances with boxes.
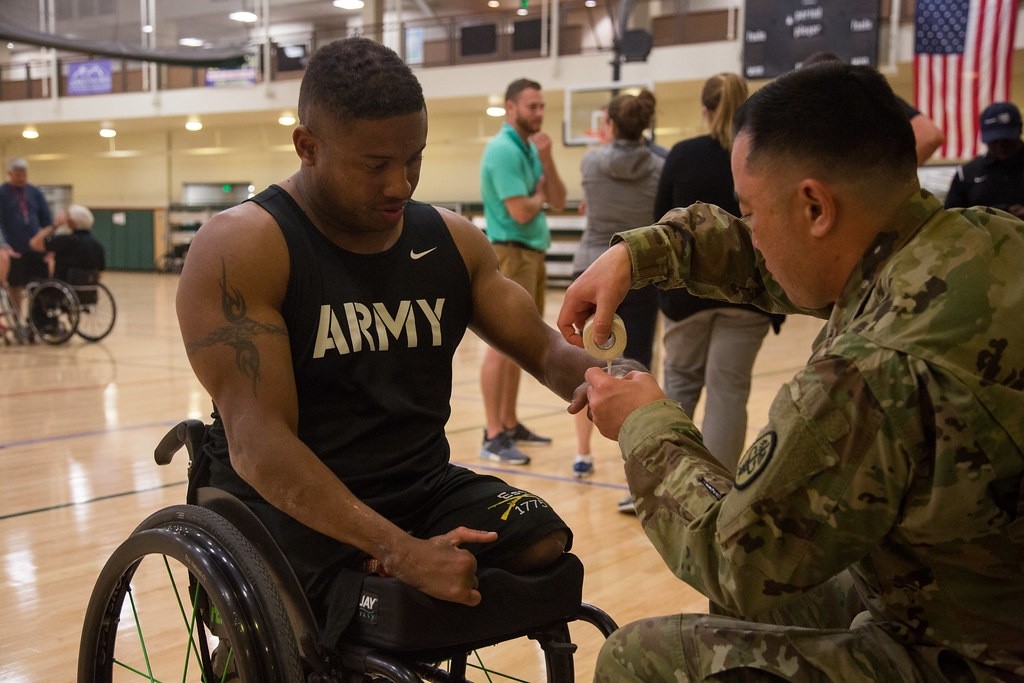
[914,0,1019,160]
[743,0,880,79]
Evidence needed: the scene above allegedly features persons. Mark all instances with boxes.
[944,102,1024,221]
[572,85,668,478]
[0,160,55,337]
[176,38,649,667]
[483,77,552,467]
[556,63,1024,683]
[26,205,106,326]
[802,50,945,168]
[618,71,786,513]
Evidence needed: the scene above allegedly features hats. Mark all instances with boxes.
[978,103,1022,143]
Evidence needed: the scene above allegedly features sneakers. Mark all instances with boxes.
[572,459,595,481]
[479,421,554,466]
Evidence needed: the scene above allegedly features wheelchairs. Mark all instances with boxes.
[4,256,119,346]
[75,392,621,683]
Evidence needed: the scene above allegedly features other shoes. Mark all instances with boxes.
[617,495,635,514]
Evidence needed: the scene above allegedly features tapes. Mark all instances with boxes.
[581,312,629,375]
